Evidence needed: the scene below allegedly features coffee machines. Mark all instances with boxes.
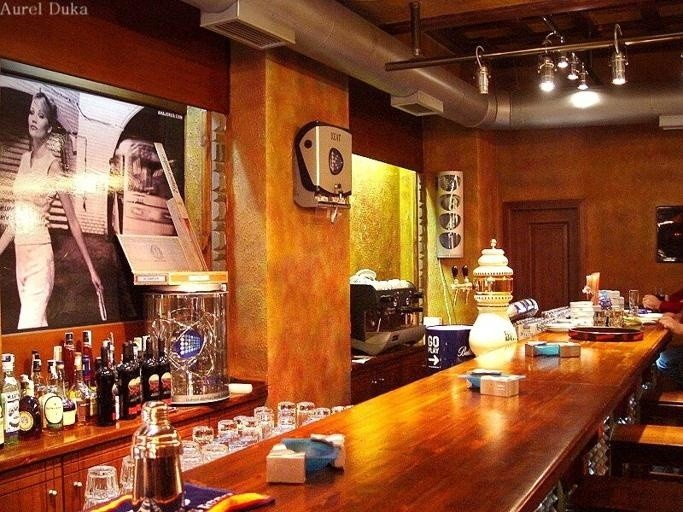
[349,277,425,353]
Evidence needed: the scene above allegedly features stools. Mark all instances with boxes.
[565,343,683,512]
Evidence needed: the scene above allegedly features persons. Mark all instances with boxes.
[656,313,682,390]
[645,287,683,312]
[0,91,105,327]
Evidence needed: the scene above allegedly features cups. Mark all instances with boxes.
[82,401,352,512]
[549,290,660,342]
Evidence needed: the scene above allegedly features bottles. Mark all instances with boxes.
[0,329,172,449]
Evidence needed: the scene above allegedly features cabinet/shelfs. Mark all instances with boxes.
[1,398,266,512]
[351,363,402,405]
[403,351,430,385]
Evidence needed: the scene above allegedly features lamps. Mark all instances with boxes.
[385,18,683,95]
